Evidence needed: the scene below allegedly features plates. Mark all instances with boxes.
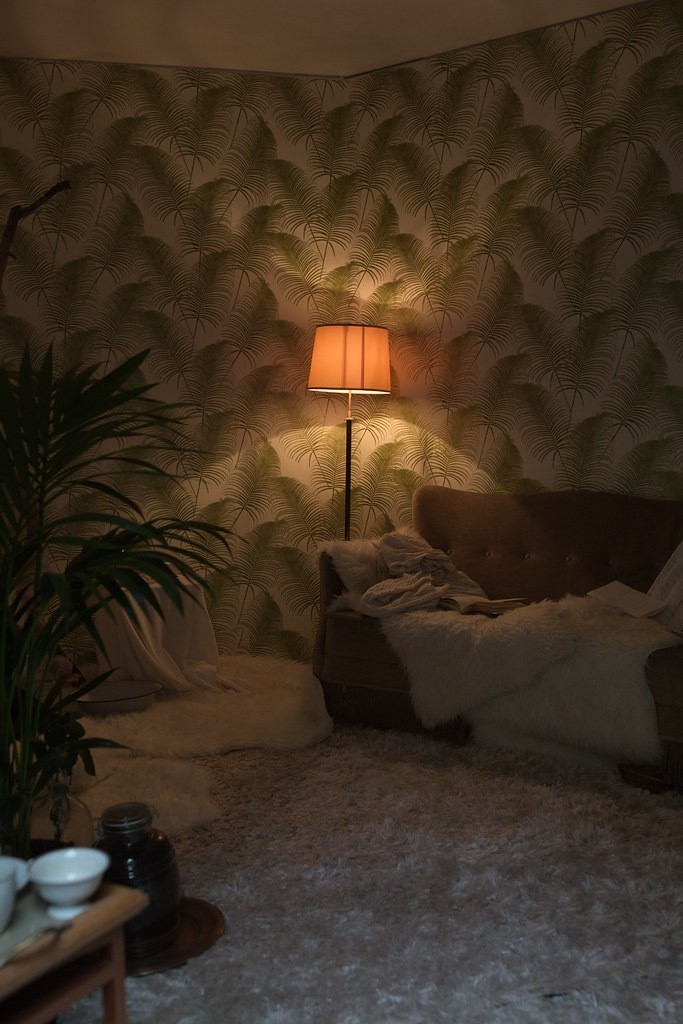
[0,855,30,891]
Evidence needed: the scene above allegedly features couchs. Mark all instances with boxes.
[311,483,683,794]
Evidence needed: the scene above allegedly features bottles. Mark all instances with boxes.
[91,801,185,980]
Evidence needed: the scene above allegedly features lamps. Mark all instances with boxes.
[305,324,392,541]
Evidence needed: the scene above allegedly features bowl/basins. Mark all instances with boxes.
[29,846,110,921]
[0,862,17,933]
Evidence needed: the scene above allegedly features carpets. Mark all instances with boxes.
[0,655,335,840]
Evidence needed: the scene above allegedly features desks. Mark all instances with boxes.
[0,882,150,1024]
